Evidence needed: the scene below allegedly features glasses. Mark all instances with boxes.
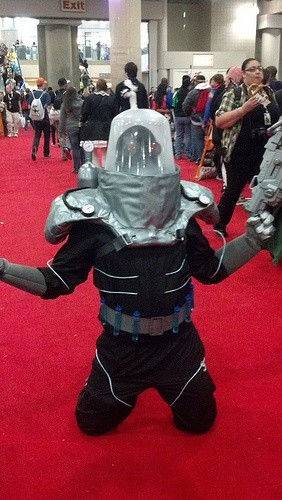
[244,66,264,72]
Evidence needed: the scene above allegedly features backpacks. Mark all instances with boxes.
[29,90,47,120]
[183,89,209,117]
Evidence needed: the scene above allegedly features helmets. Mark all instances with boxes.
[104,109,176,176]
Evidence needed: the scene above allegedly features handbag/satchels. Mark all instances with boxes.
[80,120,90,140]
[19,115,26,128]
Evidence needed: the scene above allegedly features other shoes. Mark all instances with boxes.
[236,193,245,205]
[63,147,72,160]
[214,226,227,237]
[58,143,60,147]
[8,133,13,137]
[32,146,36,160]
[63,156,67,160]
[72,169,79,173]
[51,143,55,146]
[14,133,18,138]
[222,186,227,194]
[45,155,51,157]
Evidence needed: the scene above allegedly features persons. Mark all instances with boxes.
[0,40,26,88]
[0,84,36,138]
[96,42,109,60]
[115,62,149,112]
[148,67,241,180]
[28,78,119,172]
[214,58,282,237]
[262,66,282,116]
[0,109,274,436]
[78,49,89,87]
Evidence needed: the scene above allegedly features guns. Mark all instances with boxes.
[241,114,282,246]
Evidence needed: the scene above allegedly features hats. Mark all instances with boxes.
[36,77,47,85]
[58,78,70,86]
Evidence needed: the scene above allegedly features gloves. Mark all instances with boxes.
[0,258,46,296]
[212,211,276,275]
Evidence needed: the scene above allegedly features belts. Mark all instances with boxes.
[100,303,192,336]
